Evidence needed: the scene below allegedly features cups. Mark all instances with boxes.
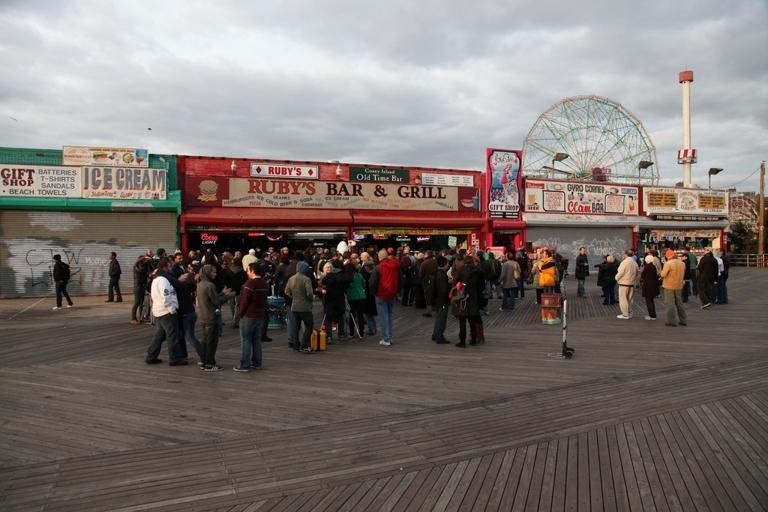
[527,192,536,205]
[472,196,478,209]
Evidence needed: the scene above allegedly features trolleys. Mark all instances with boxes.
[544,295,576,360]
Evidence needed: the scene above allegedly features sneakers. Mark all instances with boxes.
[130,320,140,324]
[261,336,272,341]
[233,366,248,372]
[645,316,655,321]
[701,302,711,309]
[617,314,630,319]
[197,361,224,372]
[169,361,188,366]
[664,322,687,326]
[422,313,432,317]
[432,333,485,347]
[288,342,315,353]
[52,307,62,310]
[380,340,391,345]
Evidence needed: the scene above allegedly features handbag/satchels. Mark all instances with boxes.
[422,272,436,305]
[514,269,521,282]
[451,295,466,318]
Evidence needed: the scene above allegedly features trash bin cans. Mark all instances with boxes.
[541,293,562,324]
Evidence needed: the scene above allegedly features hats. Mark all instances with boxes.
[665,250,675,259]
[297,262,309,273]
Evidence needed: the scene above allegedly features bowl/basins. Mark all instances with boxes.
[460,199,472,207]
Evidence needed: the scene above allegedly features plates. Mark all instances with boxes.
[122,153,133,162]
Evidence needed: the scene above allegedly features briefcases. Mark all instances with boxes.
[310,328,327,351]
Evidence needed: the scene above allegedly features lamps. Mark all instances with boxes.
[638,160,655,184]
[550,152,569,179]
[707,167,724,190]
[228,161,239,174]
[334,164,344,178]
[414,173,421,185]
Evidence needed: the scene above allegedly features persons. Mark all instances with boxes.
[104,251,123,302]
[502,163,513,195]
[627,196,635,213]
[52,254,75,309]
